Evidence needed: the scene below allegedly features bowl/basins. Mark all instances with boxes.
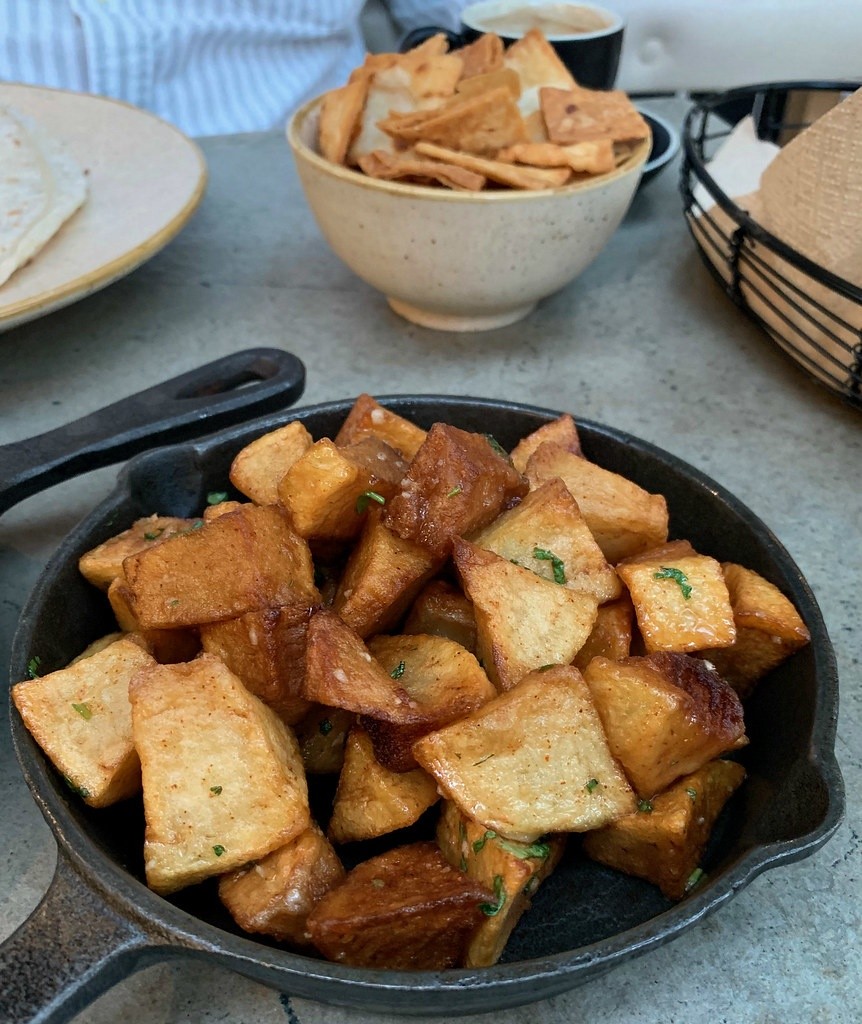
[285,83,656,334]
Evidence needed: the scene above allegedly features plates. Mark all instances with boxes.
[0,84,210,336]
[633,104,681,188]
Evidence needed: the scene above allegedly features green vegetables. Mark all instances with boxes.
[27,490,705,918]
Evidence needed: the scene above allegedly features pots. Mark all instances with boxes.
[1,392,846,1024]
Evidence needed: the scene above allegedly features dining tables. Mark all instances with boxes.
[0,95,862,1024]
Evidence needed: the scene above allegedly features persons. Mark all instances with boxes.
[0,0,470,142]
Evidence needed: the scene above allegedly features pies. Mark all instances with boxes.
[0,100,88,289]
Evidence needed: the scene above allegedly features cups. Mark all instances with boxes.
[394,1,625,92]
